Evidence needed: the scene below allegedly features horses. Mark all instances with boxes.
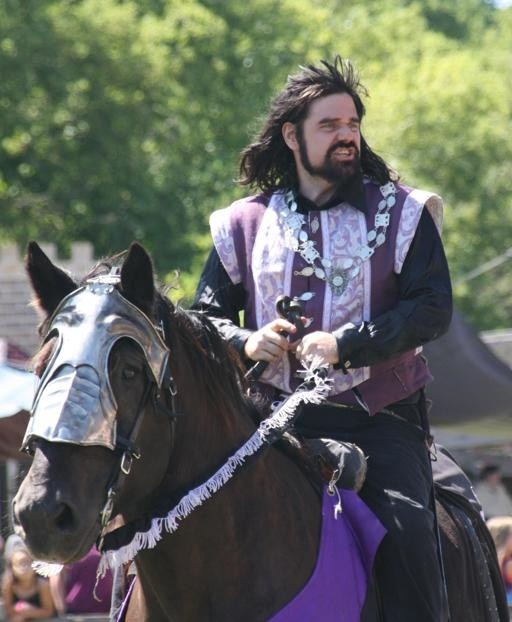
[10,240,511,622]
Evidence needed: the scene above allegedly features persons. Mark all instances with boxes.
[47,540,117,618]
[469,464,512,521]
[1,534,55,621]
[191,55,453,621]
